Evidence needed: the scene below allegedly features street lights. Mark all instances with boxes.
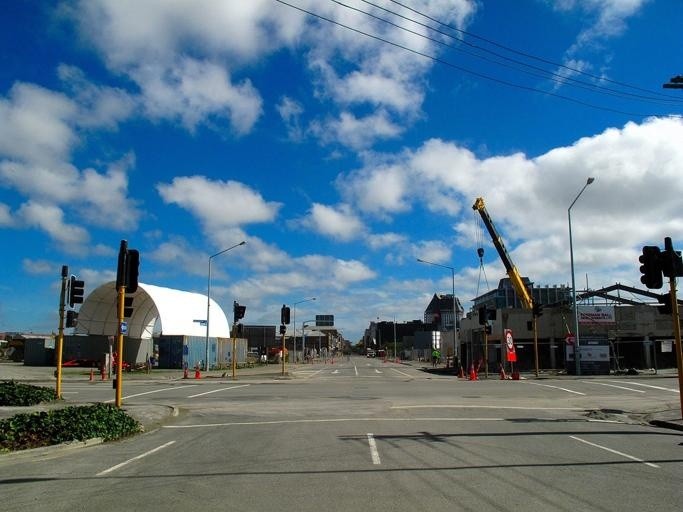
[292,297,316,364]
[566,175,595,376]
[415,258,459,375]
[376,313,396,362]
[202,239,248,372]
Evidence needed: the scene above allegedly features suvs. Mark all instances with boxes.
[243,345,290,365]
[366,351,376,358]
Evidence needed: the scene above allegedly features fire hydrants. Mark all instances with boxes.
[99,366,108,381]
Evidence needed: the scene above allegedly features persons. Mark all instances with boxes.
[431,348,438,368]
[436,348,440,364]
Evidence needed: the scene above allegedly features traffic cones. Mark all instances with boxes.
[88,368,94,383]
[457,365,465,377]
[194,365,201,379]
[469,367,475,381]
[473,366,479,380]
[182,366,190,379]
[498,365,520,381]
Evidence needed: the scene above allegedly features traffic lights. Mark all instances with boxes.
[279,325,286,334]
[64,310,80,328]
[68,275,85,308]
[237,324,244,334]
[638,244,662,289]
[235,305,246,318]
[115,295,134,319]
[531,301,544,319]
[526,320,533,331]
